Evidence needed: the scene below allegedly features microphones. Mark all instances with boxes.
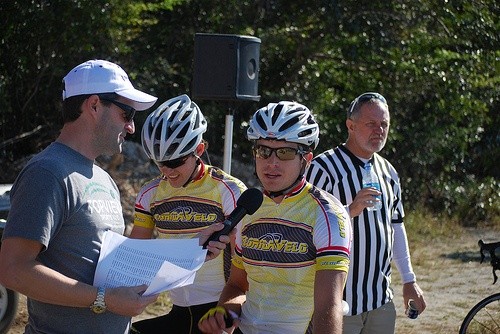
[202,188,264,254]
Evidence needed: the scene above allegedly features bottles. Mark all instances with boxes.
[362,163,382,212]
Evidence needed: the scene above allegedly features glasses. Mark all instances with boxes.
[253,144,307,161]
[150,152,193,169]
[111,99,136,123]
[350,92,388,112]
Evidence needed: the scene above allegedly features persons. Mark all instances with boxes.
[1,59,231,334]
[129,94,249,334]
[305,92,426,334]
[198,101,351,334]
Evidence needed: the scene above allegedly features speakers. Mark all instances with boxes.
[191,33,261,103]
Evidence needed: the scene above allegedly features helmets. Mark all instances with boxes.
[247,101,319,150]
[140,94,207,161]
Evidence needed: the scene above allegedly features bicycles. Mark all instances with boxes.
[0,189,20,333]
[457,238,500,334]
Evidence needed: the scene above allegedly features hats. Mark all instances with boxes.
[62,59,158,111]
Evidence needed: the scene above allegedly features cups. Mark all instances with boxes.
[404,300,419,319]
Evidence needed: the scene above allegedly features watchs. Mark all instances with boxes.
[89,286,107,314]
